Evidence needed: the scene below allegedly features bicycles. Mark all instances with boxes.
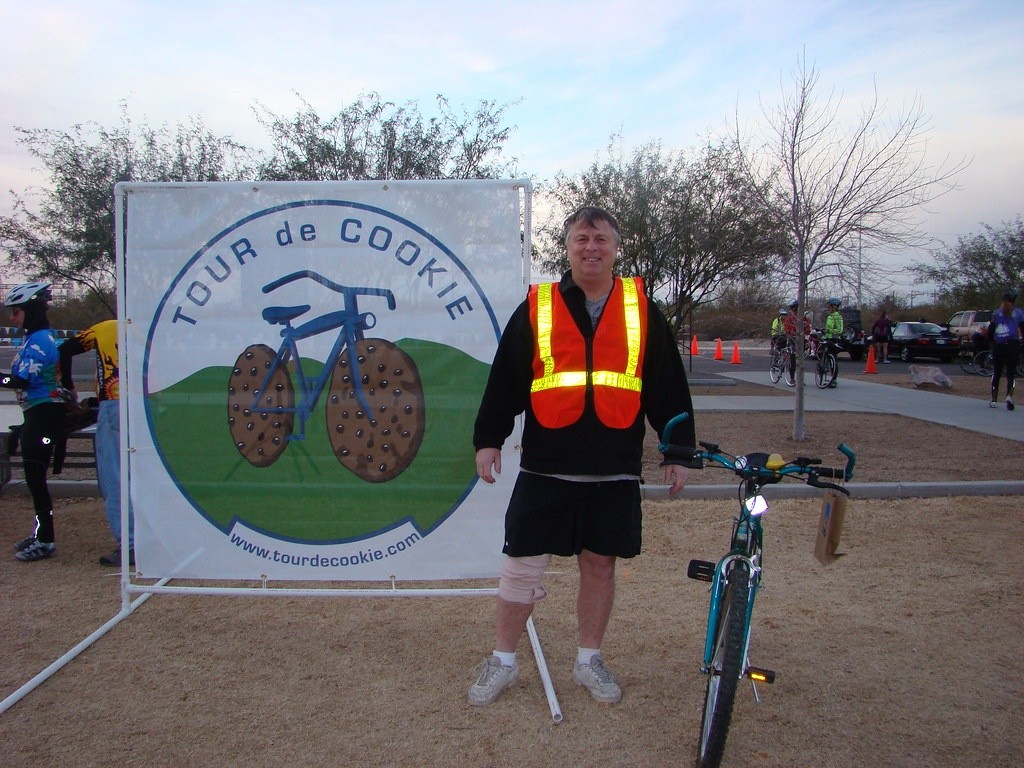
[814,328,838,389]
[770,330,812,388]
[959,338,1024,379]
[658,410,857,768]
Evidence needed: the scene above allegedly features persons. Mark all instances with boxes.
[0,282,67,561]
[871,310,898,364]
[58,320,135,567]
[771,300,813,387]
[467,206,702,706]
[822,298,843,388]
[987,291,1024,410]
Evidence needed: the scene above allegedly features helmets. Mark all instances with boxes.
[825,297,841,306]
[789,300,798,306]
[4,282,53,306]
[778,309,788,316]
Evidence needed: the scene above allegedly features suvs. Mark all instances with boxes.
[800,307,867,362]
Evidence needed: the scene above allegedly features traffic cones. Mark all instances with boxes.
[729,340,742,364]
[713,338,725,360]
[862,345,879,374]
[691,335,699,355]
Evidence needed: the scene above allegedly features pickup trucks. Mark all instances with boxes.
[937,308,993,343]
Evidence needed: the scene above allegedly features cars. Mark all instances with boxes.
[867,320,962,365]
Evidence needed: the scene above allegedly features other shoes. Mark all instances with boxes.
[875,360,879,363]
[830,382,838,388]
[883,360,891,363]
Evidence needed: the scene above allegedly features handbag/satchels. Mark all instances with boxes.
[813,466,848,568]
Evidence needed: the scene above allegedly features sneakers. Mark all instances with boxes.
[989,401,997,408]
[14,535,36,550]
[1006,396,1014,410]
[572,654,622,703]
[470,656,518,705]
[100,549,134,566]
[15,540,56,561]
